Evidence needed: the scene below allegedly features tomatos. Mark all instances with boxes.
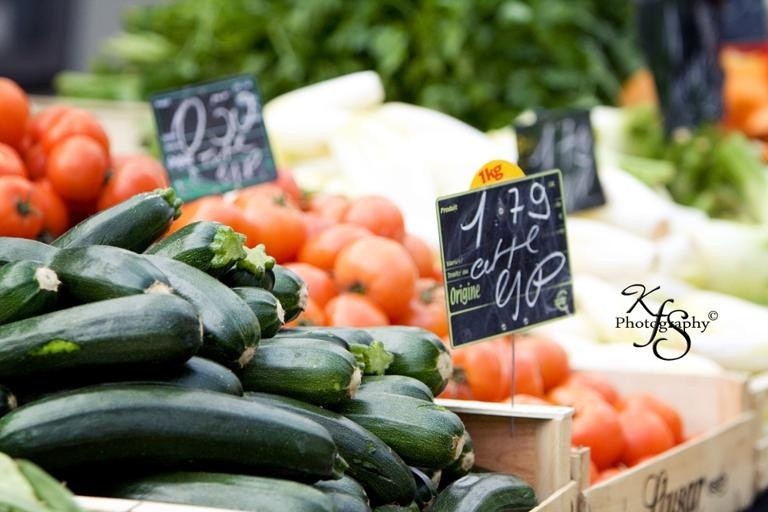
[0,74,685,485]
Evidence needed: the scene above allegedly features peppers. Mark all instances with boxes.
[52,0,768,223]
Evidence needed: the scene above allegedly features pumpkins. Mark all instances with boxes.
[0,186,537,512]
